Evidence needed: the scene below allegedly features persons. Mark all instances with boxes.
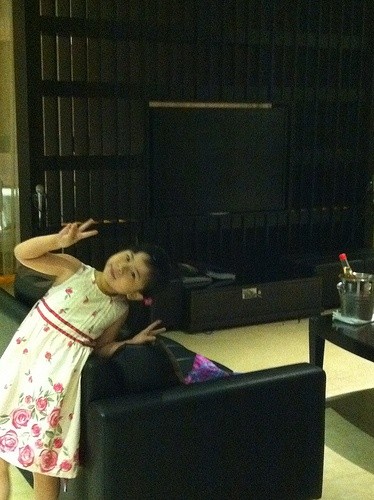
[0,219,166,500]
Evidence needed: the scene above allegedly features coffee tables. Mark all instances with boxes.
[308,315,374,368]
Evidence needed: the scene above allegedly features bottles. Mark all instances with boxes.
[339,253,356,279]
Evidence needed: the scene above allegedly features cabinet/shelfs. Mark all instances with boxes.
[144,251,374,333]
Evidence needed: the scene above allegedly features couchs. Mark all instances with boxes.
[0,271,327,500]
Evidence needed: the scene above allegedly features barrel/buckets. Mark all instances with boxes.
[337,272,374,320]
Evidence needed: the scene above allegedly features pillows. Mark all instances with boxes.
[183,356,228,384]
